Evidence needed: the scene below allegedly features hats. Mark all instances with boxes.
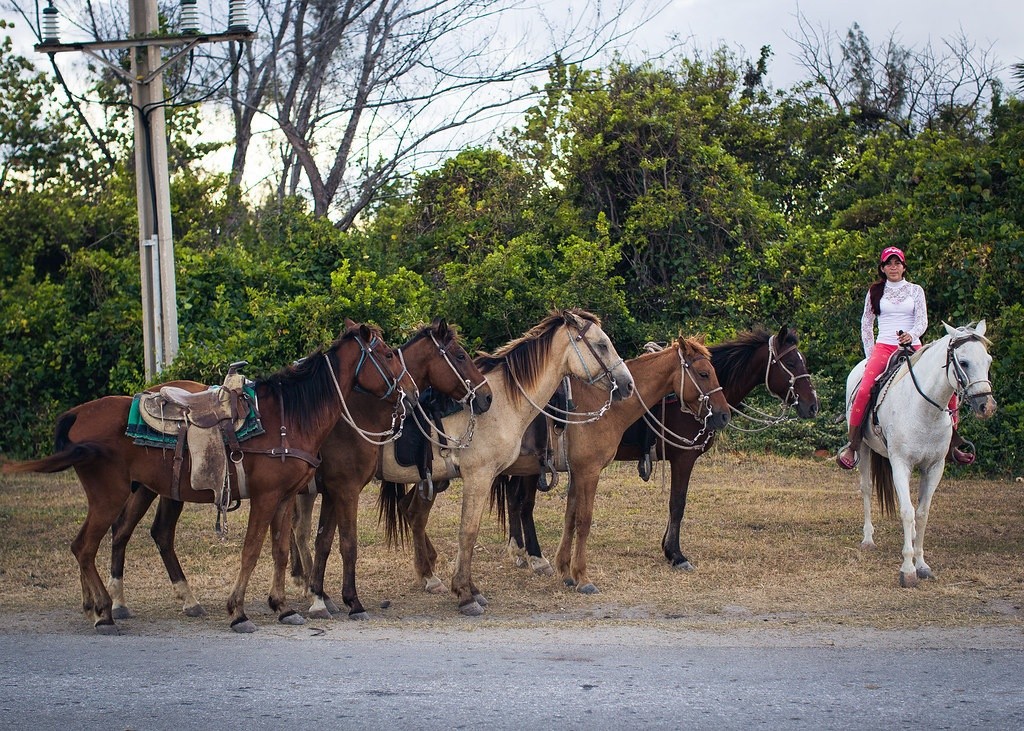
[881,246,906,265]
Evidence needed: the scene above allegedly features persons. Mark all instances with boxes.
[839,246,975,469]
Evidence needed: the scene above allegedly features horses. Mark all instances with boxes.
[0,308,817,635]
[847,319,996,587]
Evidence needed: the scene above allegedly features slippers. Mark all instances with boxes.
[948,449,975,465]
[840,452,855,469]
